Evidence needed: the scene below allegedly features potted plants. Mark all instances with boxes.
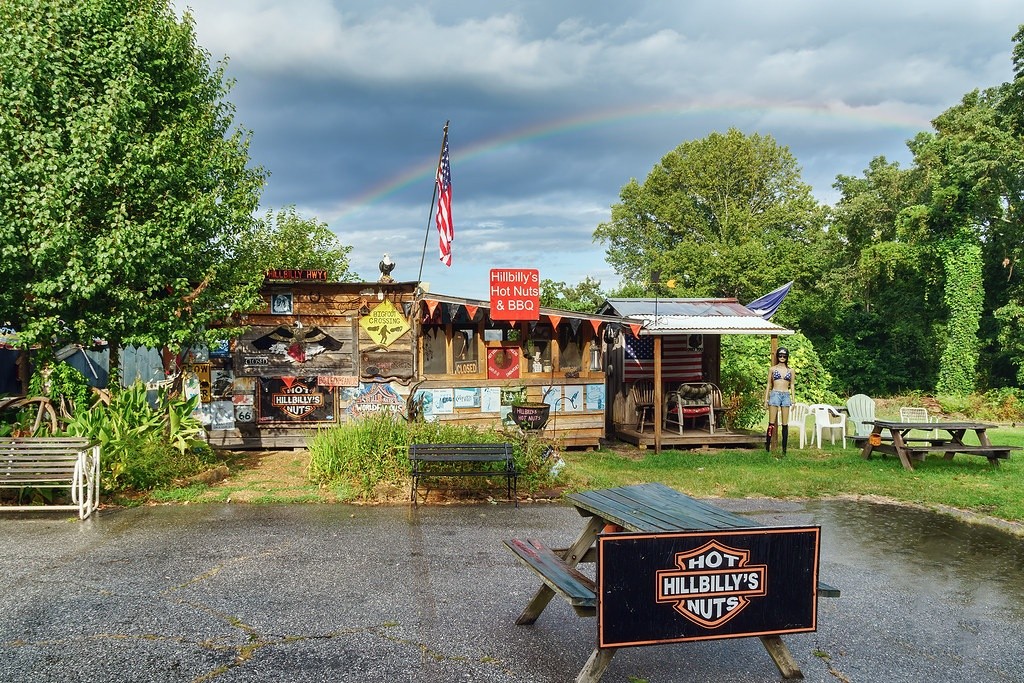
[512,356,555,429]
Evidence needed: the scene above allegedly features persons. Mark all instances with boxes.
[764,347,795,455]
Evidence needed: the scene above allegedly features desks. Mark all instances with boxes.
[811,406,847,446]
[519,481,804,683]
[861,421,1000,472]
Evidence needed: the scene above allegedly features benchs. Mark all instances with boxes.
[0,437,101,520]
[410,444,519,507]
[909,446,1020,451]
[845,434,953,442]
[503,537,599,617]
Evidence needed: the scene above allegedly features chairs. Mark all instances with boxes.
[846,394,875,434]
[900,407,939,439]
[664,383,714,435]
[809,404,846,449]
[778,403,809,449]
[632,378,655,434]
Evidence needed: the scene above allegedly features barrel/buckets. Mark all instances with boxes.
[589,345,600,370]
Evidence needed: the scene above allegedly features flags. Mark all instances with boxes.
[436,133,454,267]
[622,330,704,384]
[746,282,792,320]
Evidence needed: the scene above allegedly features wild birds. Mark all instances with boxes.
[379,253,395,276]
